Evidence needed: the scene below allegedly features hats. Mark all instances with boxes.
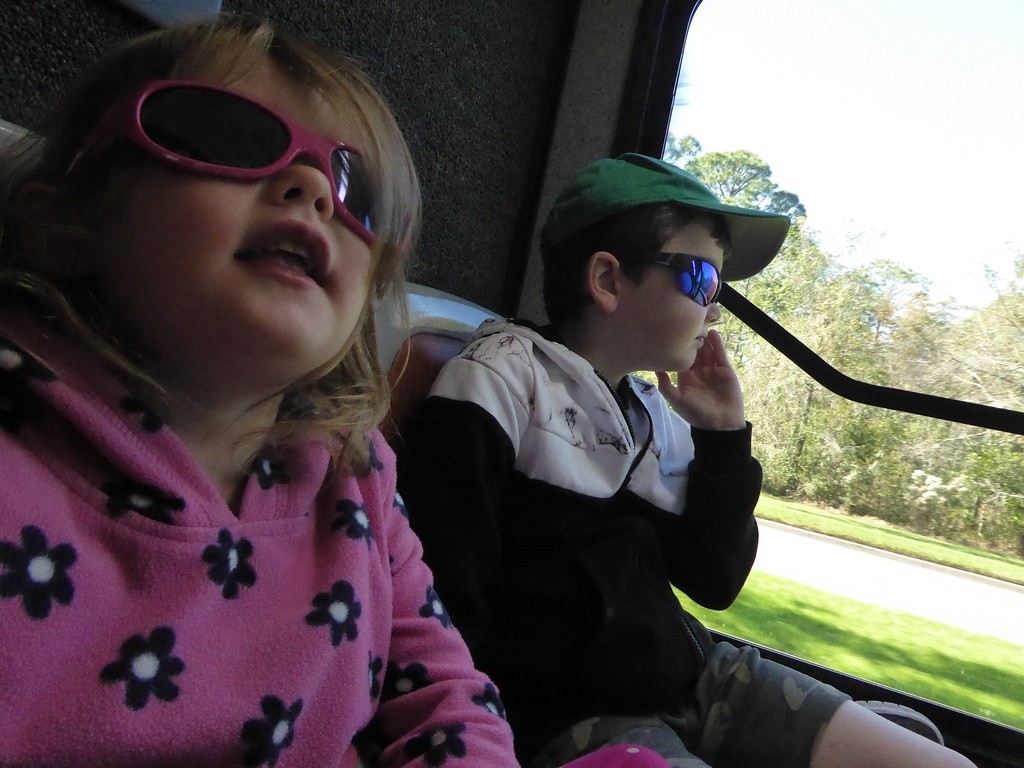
[541,152,791,282]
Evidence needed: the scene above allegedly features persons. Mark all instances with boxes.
[1,27,669,768]
[394,151,982,768]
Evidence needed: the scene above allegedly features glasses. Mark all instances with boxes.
[128,79,387,246]
[610,247,723,307]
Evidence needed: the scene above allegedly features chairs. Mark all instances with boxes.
[0,118,525,558]
